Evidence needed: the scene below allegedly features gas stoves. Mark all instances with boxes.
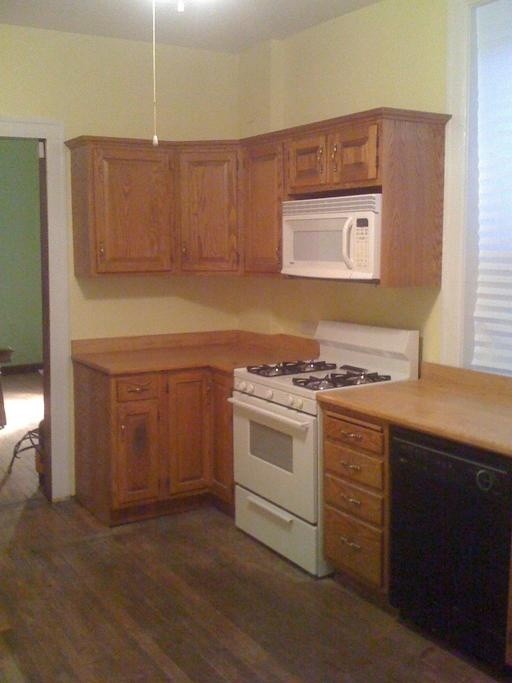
[228,319,424,408]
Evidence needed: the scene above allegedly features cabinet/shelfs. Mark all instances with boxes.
[108,368,207,527]
[320,401,390,606]
[177,141,245,279]
[283,119,383,190]
[208,372,234,515]
[242,135,287,278]
[66,136,178,285]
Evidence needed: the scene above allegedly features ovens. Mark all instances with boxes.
[223,391,330,580]
[280,193,383,283]
